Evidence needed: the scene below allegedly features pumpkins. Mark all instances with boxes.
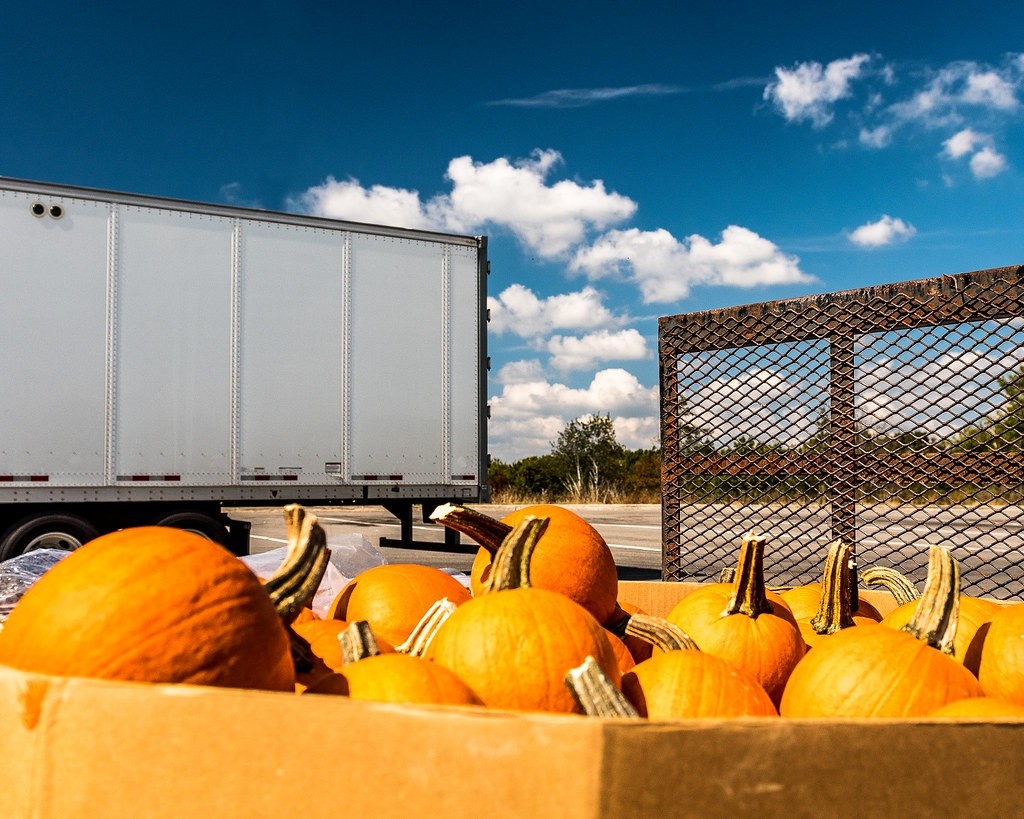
[0,501,1024,723]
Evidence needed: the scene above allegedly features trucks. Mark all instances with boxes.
[0,176,495,558]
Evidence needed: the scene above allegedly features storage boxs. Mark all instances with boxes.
[0,583,1024,819]
